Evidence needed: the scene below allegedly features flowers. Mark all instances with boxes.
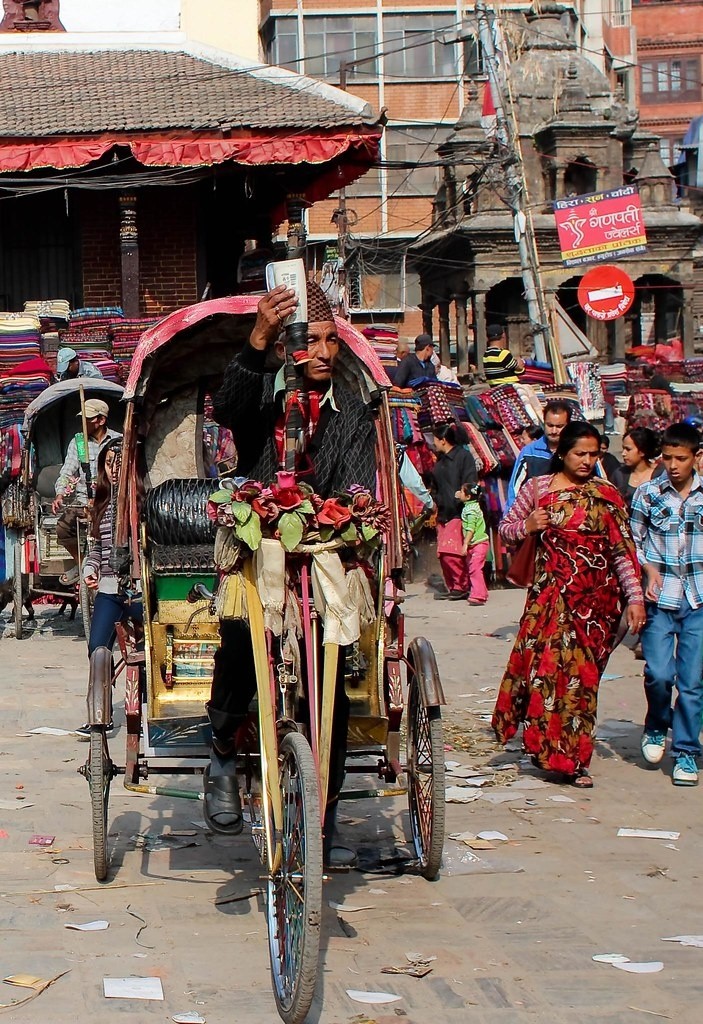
[207,471,394,552]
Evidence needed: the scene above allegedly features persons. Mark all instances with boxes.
[56,348,102,381]
[394,335,436,388]
[641,366,673,393]
[203,278,378,872]
[483,324,525,390]
[628,422,703,786]
[499,421,646,789]
[430,350,460,386]
[75,437,146,737]
[51,399,124,586]
[429,400,702,608]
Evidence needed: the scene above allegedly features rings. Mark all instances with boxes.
[273,306,280,314]
[276,314,282,321]
[638,621,642,624]
[642,621,645,625]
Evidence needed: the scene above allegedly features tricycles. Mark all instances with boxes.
[79,294,449,1024]
[10,378,127,640]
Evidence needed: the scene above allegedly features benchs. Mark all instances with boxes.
[143,479,220,604]
[35,465,78,521]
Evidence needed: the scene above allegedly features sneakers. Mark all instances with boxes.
[640,725,667,765]
[672,754,699,785]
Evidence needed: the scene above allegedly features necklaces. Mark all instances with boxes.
[550,472,585,526]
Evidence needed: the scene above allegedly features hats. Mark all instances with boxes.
[486,324,503,341]
[57,348,76,372]
[415,334,439,351]
[684,416,702,428]
[274,281,335,334]
[77,399,109,417]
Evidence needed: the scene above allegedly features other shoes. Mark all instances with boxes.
[466,598,486,605]
[75,717,114,737]
[140,720,145,737]
[634,643,646,660]
[565,768,594,787]
[605,424,621,435]
[448,589,468,599]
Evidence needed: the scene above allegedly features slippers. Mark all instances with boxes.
[59,565,82,585]
[203,762,245,836]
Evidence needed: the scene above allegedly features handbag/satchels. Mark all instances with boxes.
[504,477,537,588]
